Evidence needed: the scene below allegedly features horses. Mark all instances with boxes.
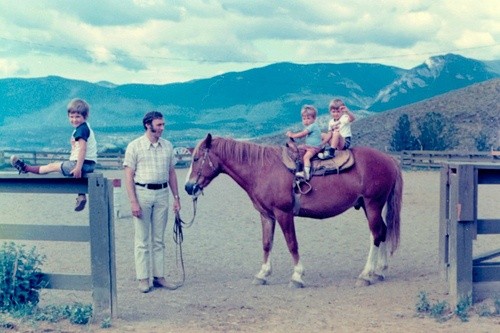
[185,133,404,290]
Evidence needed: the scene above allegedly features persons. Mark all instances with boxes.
[10,98,98,212]
[286,104,323,180]
[122,110,182,294]
[318,97,357,160]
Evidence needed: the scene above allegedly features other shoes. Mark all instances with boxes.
[10,155,27,174]
[153,277,177,290]
[74,194,87,211]
[318,150,335,159]
[139,277,150,292]
[296,171,310,179]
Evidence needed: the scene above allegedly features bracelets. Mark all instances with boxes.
[174,195,182,199]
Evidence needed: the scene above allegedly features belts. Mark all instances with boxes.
[135,182,168,190]
